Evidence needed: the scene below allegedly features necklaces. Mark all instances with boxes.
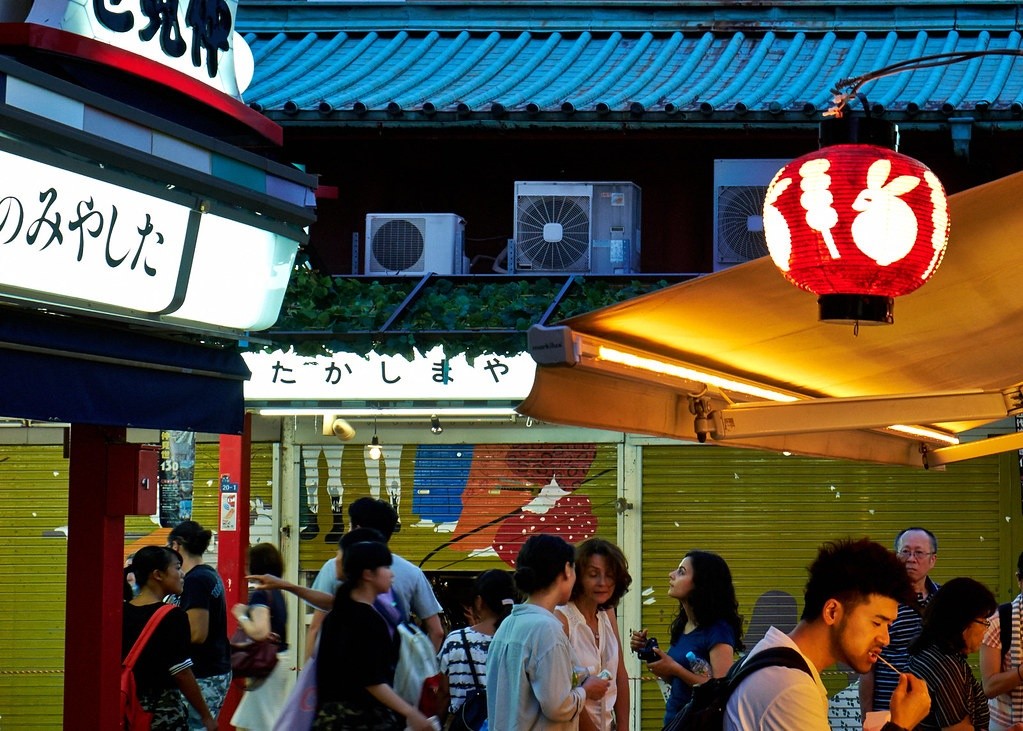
[591,628,599,640]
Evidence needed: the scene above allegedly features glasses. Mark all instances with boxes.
[895,548,936,560]
[973,618,990,631]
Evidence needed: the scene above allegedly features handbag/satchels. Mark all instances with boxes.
[229,629,279,677]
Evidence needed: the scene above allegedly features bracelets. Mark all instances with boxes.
[1018,665,1023,681]
[880,721,908,731]
[238,616,248,619]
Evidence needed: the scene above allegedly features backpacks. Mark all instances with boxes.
[375,589,450,726]
[449,627,490,731]
[663,644,816,731]
[119,602,176,731]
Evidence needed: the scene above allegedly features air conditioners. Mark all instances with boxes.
[511,180,641,273]
[712,158,798,272]
[365,212,467,275]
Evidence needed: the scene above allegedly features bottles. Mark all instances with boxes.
[570,665,597,688]
[685,651,712,679]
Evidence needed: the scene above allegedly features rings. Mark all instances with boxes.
[649,668,653,671]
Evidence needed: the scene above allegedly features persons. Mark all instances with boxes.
[124,546,217,731]
[486,534,611,731]
[245,529,400,731]
[979,553,1023,731]
[436,568,521,731]
[552,537,632,731]
[305,497,444,663]
[168,521,232,731]
[229,543,291,731]
[724,535,932,731]
[310,541,435,731]
[629,550,745,731]
[859,526,941,724]
[903,577,1023,731]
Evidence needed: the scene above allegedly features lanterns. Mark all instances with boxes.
[763,143,950,327]
